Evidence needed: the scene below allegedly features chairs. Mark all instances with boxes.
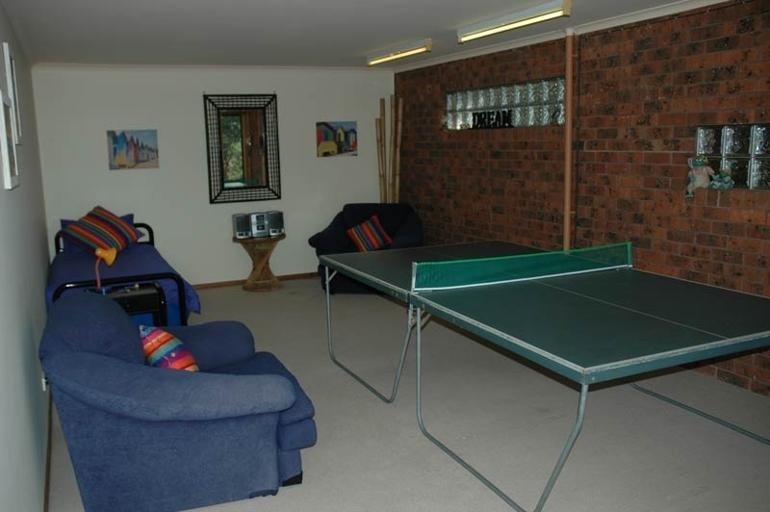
[306,203,424,294]
[39,288,317,512]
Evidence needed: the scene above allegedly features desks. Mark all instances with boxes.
[233,234,285,290]
[318,239,770,512]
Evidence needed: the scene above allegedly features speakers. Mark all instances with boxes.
[230,211,285,240]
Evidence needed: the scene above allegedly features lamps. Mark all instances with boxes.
[457,9,571,44]
[368,46,431,67]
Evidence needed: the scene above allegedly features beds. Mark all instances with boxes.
[44,221,200,325]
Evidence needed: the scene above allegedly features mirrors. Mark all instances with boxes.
[203,95,281,203]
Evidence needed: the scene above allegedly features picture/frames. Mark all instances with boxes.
[0,88,21,192]
[105,125,162,173]
[4,41,23,143]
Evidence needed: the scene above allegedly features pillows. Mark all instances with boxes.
[61,214,136,259]
[347,213,392,252]
[138,324,199,371]
[60,205,145,262]
